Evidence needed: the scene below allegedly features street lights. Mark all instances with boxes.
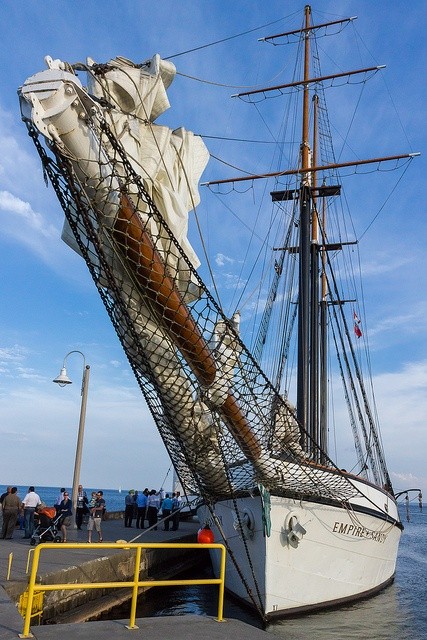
[52,347,90,526]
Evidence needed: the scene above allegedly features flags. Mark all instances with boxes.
[354,311,362,338]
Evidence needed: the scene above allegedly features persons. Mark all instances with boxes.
[20,484,41,539]
[176,491,182,529]
[57,491,72,543]
[0,486,13,514]
[0,486,21,540]
[87,491,105,543]
[170,492,177,531]
[90,492,97,518]
[135,490,148,529]
[55,488,71,510]
[132,490,139,519]
[76,485,87,530]
[125,490,134,527]
[161,494,172,532]
[146,490,160,531]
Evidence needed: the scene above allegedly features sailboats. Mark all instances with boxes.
[154,462,204,520]
[18,5,422,622]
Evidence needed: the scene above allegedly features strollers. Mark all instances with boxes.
[30,505,62,546]
